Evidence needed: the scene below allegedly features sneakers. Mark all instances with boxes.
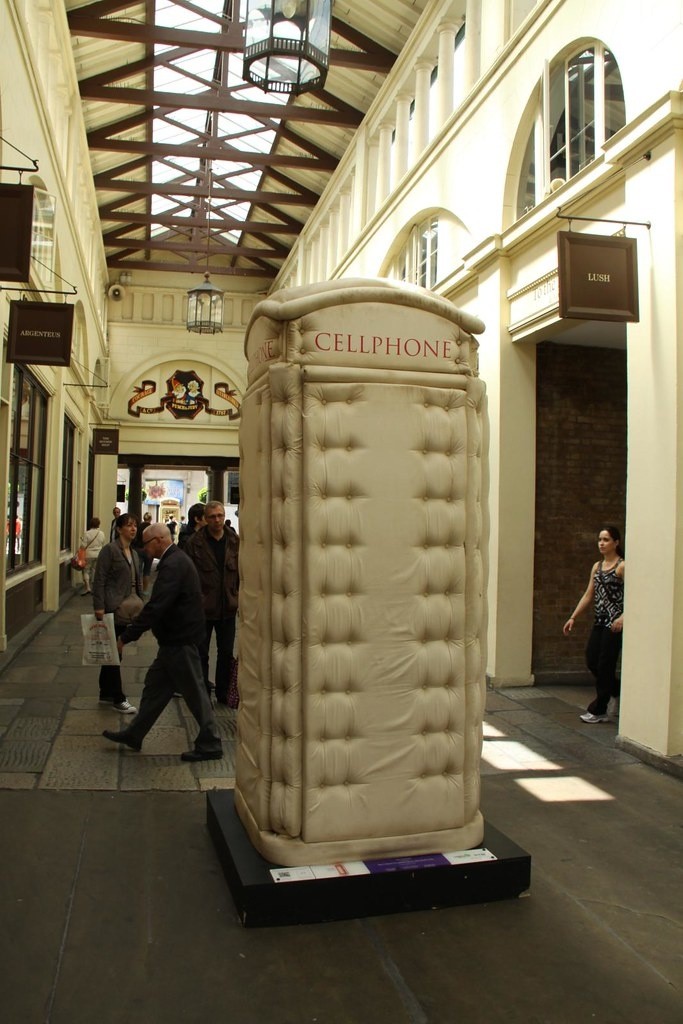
[113,697,137,714]
[580,712,609,723]
[611,705,619,715]
[99,698,114,704]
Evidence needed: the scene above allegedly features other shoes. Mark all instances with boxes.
[210,685,215,691]
[173,691,182,697]
[80,588,92,596]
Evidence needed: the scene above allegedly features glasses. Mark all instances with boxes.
[113,512,119,513]
[207,514,225,519]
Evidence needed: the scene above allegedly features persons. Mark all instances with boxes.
[80,517,105,597]
[92,513,143,714]
[178,503,209,546]
[183,501,239,705]
[139,512,151,595]
[564,525,625,723]
[225,520,236,534]
[110,508,121,542]
[102,523,223,761]
[165,515,187,545]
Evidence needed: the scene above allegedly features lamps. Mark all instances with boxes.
[241,1,332,97]
[186,159,225,336]
[119,272,133,287]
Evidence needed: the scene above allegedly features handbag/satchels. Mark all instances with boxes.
[115,587,145,626]
[226,652,240,708]
[70,547,86,570]
[81,612,122,666]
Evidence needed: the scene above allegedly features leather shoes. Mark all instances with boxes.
[181,748,223,761]
[102,729,142,751]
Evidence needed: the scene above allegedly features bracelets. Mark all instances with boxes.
[569,617,575,620]
